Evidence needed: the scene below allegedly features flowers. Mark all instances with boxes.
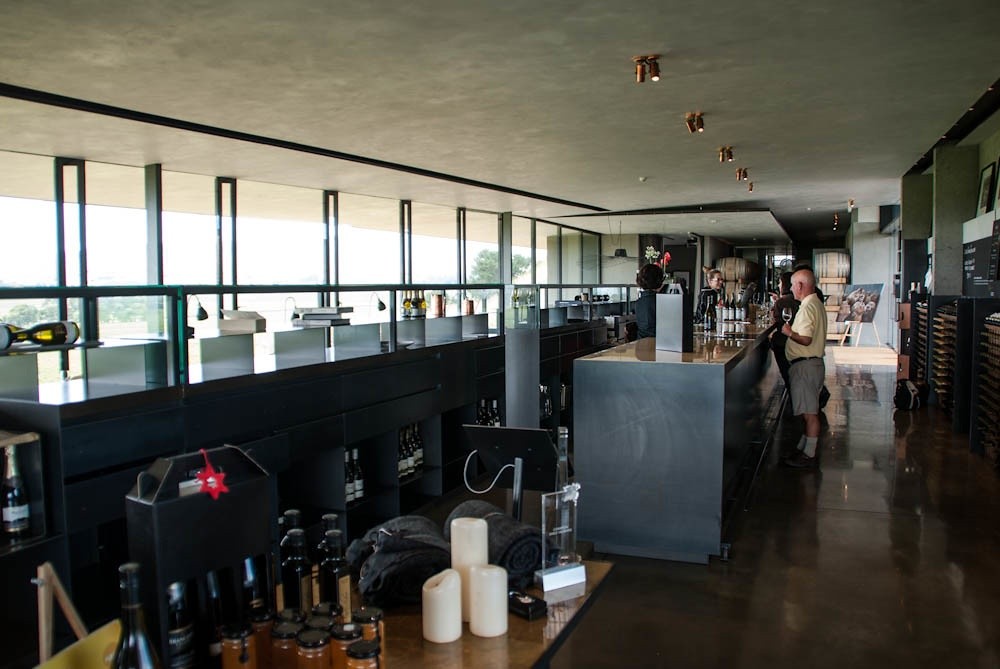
[645,246,661,261]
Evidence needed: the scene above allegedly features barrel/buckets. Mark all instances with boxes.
[813,252,850,333]
[717,257,749,302]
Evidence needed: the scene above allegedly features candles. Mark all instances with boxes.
[422,568,463,643]
[451,518,489,644]
[470,563,508,638]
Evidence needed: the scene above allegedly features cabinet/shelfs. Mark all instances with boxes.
[0,314,639,669]
[908,289,1000,474]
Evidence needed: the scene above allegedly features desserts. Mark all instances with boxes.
[836,287,880,322]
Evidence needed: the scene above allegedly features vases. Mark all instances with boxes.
[650,257,653,264]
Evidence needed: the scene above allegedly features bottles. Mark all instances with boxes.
[703,292,749,330]
[397,423,425,483]
[0,321,80,350]
[401,290,427,320]
[473,399,501,428]
[716,323,745,348]
[0,444,32,549]
[163,582,197,669]
[204,509,384,669]
[343,448,365,506]
[109,561,162,669]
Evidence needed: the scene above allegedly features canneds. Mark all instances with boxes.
[346,639,381,669]
[220,621,256,669]
[296,630,330,669]
[272,622,305,669]
[277,608,308,624]
[312,602,343,621]
[330,622,363,669]
[305,615,337,632]
[352,608,385,669]
[249,608,275,662]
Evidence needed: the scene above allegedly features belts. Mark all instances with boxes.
[790,356,817,364]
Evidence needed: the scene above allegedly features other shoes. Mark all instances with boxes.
[781,447,818,466]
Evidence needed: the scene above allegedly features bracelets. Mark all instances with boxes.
[789,332,795,340]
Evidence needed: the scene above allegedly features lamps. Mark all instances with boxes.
[187,294,208,320]
[369,293,386,324]
[833,213,838,231]
[749,183,753,191]
[735,168,748,182]
[851,200,854,205]
[615,221,627,257]
[718,146,734,162]
[684,112,704,134]
[632,55,660,83]
[282,297,300,325]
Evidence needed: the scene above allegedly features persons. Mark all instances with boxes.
[636,263,829,471]
